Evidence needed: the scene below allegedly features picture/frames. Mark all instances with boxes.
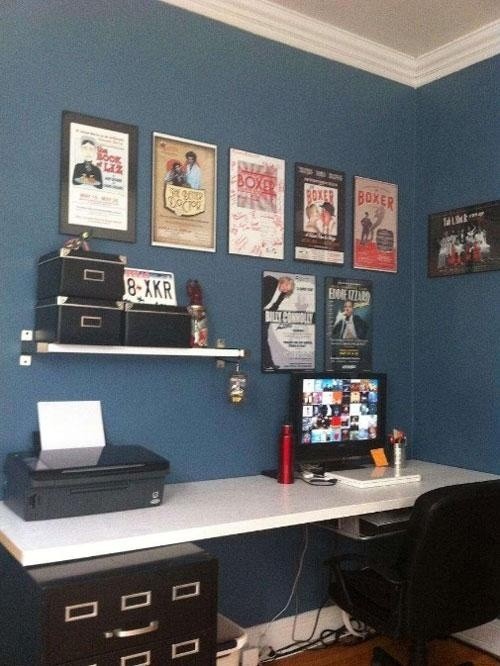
[59,109,139,244]
[428,200,500,279]
[150,130,218,255]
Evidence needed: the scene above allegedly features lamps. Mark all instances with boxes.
[217,358,249,403]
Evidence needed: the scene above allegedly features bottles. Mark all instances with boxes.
[187,305,210,347]
[277,423,295,485]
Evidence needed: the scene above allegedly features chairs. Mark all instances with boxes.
[323,478,500,664]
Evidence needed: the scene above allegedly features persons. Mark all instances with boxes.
[333,300,368,339]
[320,202,337,240]
[165,162,189,187]
[360,212,372,243]
[73,136,102,188]
[185,151,202,188]
[265,275,295,371]
[435,216,493,267]
[305,204,321,232]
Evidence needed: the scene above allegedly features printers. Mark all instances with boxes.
[3,430,169,521]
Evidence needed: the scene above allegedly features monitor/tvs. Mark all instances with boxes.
[292,372,386,469]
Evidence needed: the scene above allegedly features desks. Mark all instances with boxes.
[1,457,499,661]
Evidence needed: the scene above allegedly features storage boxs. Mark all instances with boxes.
[37,249,126,300]
[125,302,190,346]
[33,299,123,344]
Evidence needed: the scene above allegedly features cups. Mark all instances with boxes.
[392,441,408,471]
